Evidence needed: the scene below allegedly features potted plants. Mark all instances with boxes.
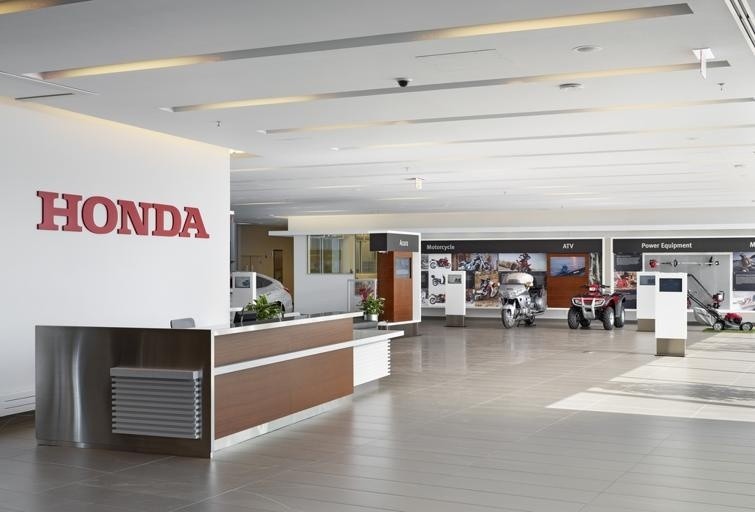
[355,294,387,322]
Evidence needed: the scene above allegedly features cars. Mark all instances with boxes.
[229,271,292,313]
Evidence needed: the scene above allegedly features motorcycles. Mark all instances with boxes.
[470,280,500,304]
[510,257,532,272]
[430,257,450,269]
[458,253,492,275]
[499,271,548,329]
[429,293,445,304]
[431,274,445,286]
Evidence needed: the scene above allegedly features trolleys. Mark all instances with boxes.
[687,273,753,331]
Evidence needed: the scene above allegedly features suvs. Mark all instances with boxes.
[568,281,625,329]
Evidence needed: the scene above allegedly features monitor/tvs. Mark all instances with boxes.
[396,257,410,278]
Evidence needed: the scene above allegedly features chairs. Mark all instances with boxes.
[171,318,194,329]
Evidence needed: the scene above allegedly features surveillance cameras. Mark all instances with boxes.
[398,80,407,87]
